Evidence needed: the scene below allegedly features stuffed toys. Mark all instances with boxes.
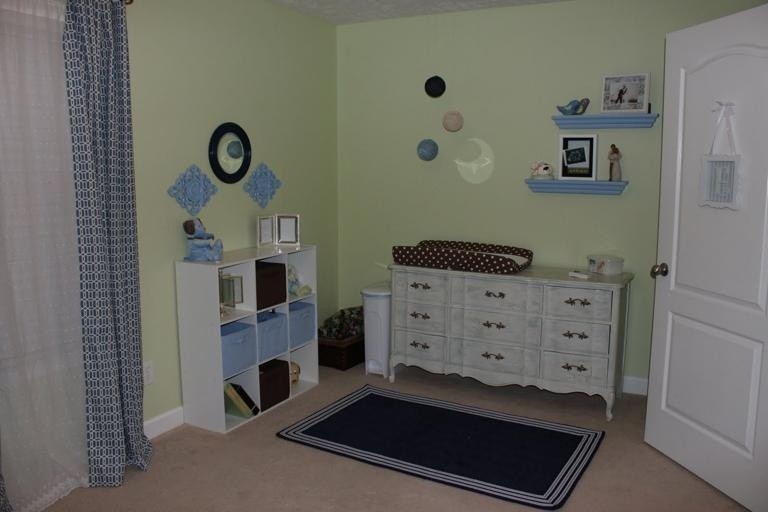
[183,218,223,261]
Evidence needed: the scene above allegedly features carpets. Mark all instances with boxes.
[274,382,607,511]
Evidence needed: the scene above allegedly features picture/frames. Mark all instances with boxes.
[256,215,275,248]
[207,121,253,185]
[557,133,598,181]
[274,213,301,254]
[600,71,651,115]
[697,154,741,211]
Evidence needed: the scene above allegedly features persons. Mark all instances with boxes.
[611,147,621,181]
[609,144,616,181]
[615,85,627,104]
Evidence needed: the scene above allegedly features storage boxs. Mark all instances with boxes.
[219,260,316,413]
[318,334,365,372]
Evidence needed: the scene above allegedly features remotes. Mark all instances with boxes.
[568,270,592,280]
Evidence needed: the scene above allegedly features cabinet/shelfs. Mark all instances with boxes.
[387,263,637,423]
[173,243,321,435]
